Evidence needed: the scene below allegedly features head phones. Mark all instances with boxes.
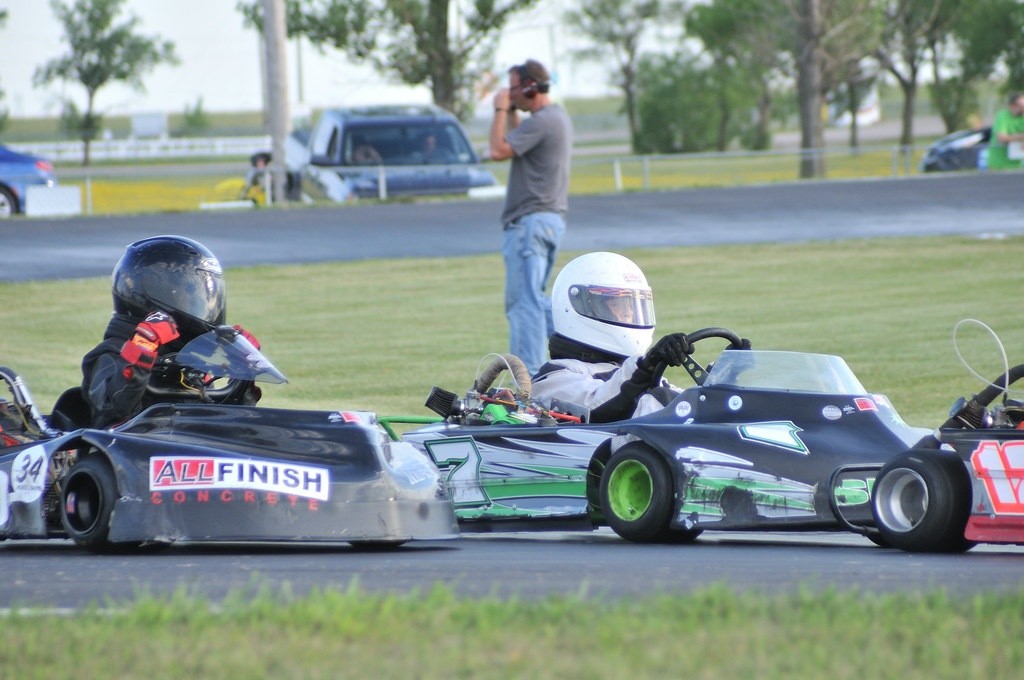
[518,66,540,99]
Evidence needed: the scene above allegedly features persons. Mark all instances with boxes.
[490,60,575,380]
[411,132,438,158]
[529,251,753,426]
[987,91,1024,170]
[80,235,262,431]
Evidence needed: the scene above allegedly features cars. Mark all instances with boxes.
[284,102,499,204]
[924,125,993,173]
[0,144,59,216]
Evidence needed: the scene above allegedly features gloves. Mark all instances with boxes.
[132,310,178,352]
[726,338,753,377]
[233,324,260,350]
[648,333,696,367]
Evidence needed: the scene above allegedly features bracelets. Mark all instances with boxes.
[496,107,508,113]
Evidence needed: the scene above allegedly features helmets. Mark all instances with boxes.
[110,234,227,347]
[552,251,656,358]
